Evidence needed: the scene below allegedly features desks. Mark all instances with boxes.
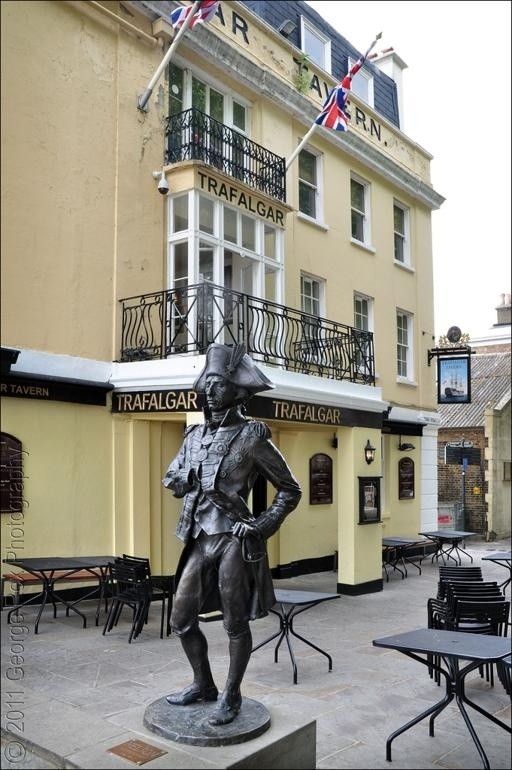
[243,587,345,684]
[418,531,477,566]
[382,537,426,583]
[373,629,512,770]
[3,556,117,635]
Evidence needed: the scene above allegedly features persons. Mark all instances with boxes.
[162,343,302,725]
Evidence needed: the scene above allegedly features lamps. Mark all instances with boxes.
[365,440,376,465]
[400,444,414,451]
[279,19,297,38]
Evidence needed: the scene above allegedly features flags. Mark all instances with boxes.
[315,40,377,131]
[171,0,219,32]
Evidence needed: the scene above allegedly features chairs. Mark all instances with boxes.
[424,564,510,690]
[103,555,178,644]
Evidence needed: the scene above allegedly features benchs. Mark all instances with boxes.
[334,540,437,572]
[0,570,116,616]
